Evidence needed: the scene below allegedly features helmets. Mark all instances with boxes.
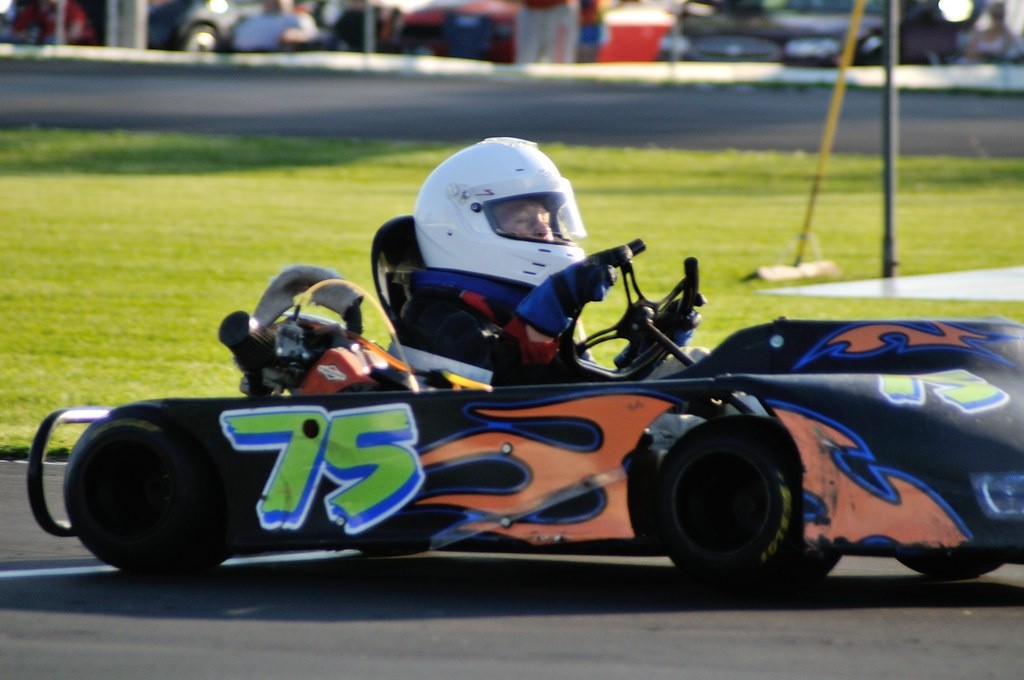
[414,137,586,287]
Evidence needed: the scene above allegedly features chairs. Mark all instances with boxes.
[371,216,453,392]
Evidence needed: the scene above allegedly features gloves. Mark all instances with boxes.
[513,245,633,339]
[614,292,706,381]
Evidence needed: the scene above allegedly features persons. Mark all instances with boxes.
[10,0,1024,69]
[379,138,703,394]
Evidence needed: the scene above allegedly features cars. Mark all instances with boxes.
[149,1,381,54]
[384,1,678,66]
[653,0,963,67]
